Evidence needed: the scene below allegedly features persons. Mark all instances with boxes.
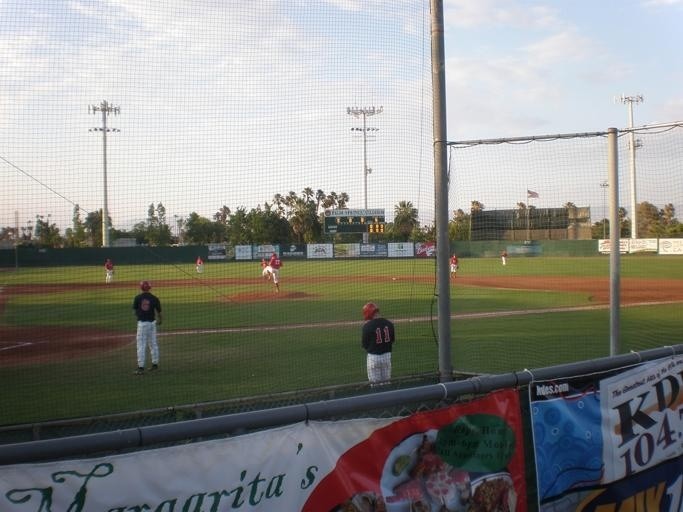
[197,256,205,274]
[261,258,271,281]
[450,254,459,278]
[360,298,395,388]
[263,252,281,294]
[103,256,114,285]
[500,247,508,267]
[132,280,161,377]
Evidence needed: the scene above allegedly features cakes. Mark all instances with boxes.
[426,472,456,504]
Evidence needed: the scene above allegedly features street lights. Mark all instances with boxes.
[88,100,122,245]
[346,104,384,209]
[599,92,644,238]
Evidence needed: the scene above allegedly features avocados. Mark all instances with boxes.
[434,412,518,474]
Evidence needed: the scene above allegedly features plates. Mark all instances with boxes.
[380,429,444,512]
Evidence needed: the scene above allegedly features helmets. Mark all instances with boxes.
[363,303,378,319]
[140,281,151,291]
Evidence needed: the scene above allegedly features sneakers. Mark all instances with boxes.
[134,368,143,374]
[148,364,158,371]
